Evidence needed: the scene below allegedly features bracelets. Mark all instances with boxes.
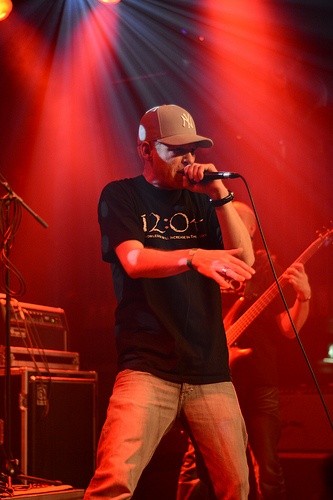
[188,248,199,271]
[297,295,312,301]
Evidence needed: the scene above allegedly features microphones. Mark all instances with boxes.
[185,170,240,184]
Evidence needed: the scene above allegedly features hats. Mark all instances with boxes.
[136,103,213,147]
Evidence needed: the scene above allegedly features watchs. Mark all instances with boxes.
[208,189,235,206]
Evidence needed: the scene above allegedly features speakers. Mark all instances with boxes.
[0,368,95,486]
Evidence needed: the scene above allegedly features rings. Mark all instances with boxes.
[222,267,228,275]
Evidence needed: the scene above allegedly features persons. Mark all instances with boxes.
[176,202,310,500]
[82,105,255,500]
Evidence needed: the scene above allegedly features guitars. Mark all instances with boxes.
[222,226,333,369]
